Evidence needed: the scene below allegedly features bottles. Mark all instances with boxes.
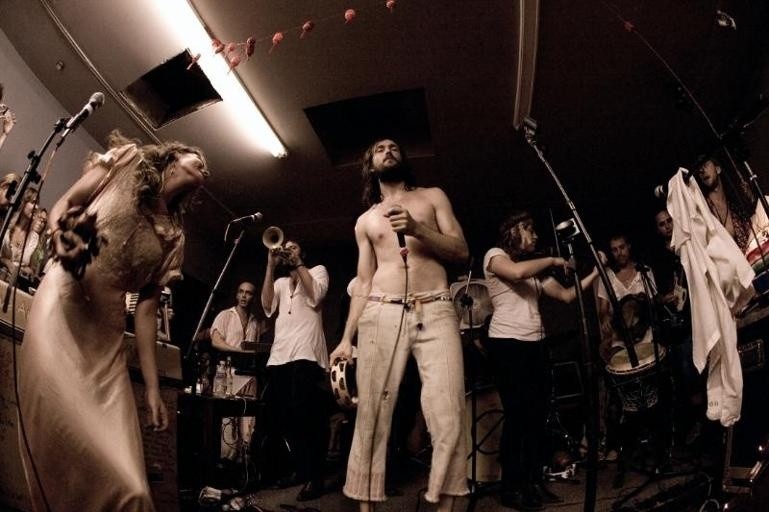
[211,359,228,397]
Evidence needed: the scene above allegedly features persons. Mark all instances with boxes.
[261,238,337,502]
[126,287,173,330]
[0,103,16,145]
[209,281,262,458]
[15,140,210,511]
[330,139,469,511]
[452,154,757,510]
[0,173,48,276]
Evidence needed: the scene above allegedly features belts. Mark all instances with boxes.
[366,296,453,305]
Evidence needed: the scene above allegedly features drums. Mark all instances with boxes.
[605,342,665,412]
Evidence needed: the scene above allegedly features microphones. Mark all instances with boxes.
[388,205,406,248]
[65,92,105,132]
[654,171,694,196]
[238,211,264,223]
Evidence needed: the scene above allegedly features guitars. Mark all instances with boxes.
[663,267,687,322]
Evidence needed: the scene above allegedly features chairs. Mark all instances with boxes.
[551,362,583,427]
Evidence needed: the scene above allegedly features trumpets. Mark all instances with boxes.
[262,227,295,268]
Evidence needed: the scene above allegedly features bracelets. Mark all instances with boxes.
[52,228,62,239]
[296,264,305,268]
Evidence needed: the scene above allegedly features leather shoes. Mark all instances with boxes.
[501,490,545,512]
[296,479,325,500]
[526,483,560,503]
